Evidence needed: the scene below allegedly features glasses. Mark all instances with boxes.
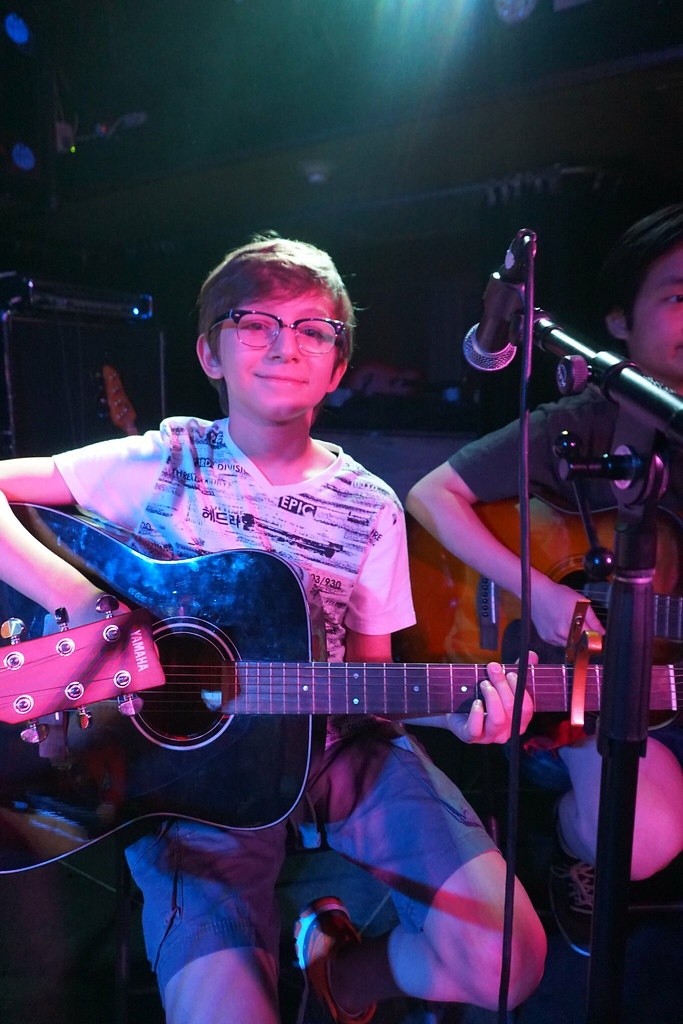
[207,309,350,360]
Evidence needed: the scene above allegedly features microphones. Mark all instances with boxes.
[463,228,537,370]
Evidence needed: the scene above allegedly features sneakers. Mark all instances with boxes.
[545,799,596,957]
[294,897,378,1024]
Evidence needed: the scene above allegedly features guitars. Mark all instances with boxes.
[0,499,681,876]
[394,489,679,734]
[93,361,138,437]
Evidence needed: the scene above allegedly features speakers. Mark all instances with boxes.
[0,308,167,461]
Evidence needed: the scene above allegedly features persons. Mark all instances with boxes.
[405,204,683,956]
[0,237,547,1024]
